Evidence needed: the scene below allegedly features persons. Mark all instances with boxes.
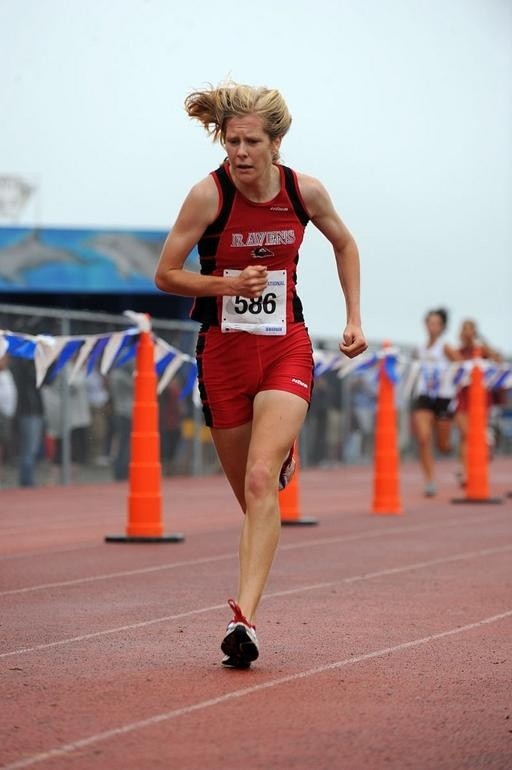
[453,321,503,486]
[155,85,368,669]
[409,310,464,496]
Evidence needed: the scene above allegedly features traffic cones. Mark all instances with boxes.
[277,431,318,528]
[452,345,502,504]
[104,313,186,543]
[370,340,405,516]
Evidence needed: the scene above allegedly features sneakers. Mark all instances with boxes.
[221,599,260,668]
[278,442,296,491]
